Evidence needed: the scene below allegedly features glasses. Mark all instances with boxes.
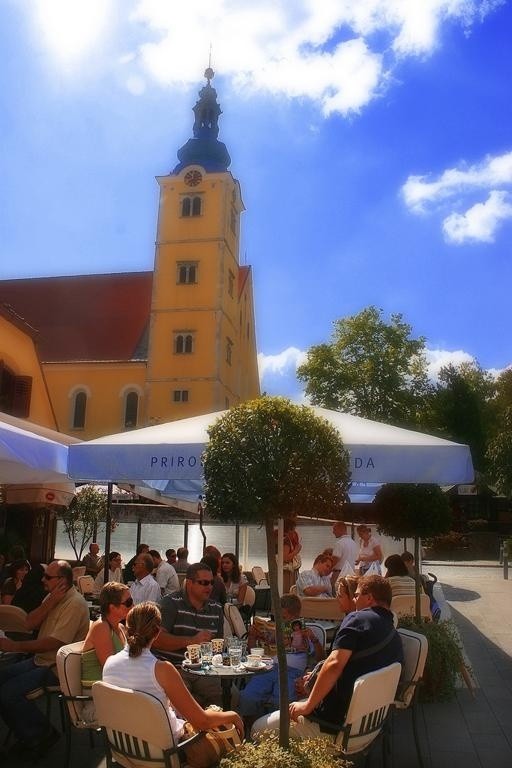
[133,562,144,567]
[195,580,215,586]
[171,554,176,558]
[119,595,133,608]
[39,571,65,581]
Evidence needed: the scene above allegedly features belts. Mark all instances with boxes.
[334,570,341,572]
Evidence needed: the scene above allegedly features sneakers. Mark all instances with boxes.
[7,724,63,762]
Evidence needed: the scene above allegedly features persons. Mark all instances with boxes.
[0,518,431,743]
[98,600,246,754]
[0,559,91,758]
[251,574,403,750]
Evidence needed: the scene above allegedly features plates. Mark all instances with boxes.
[182,659,201,668]
[242,661,267,668]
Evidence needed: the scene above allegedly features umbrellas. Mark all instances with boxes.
[65,394,478,597]
[0,410,85,526]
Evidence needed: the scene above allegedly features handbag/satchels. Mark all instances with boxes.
[181,704,245,768]
[426,581,441,624]
[303,659,345,721]
[275,535,301,573]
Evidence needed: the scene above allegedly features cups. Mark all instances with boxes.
[183,638,265,669]
